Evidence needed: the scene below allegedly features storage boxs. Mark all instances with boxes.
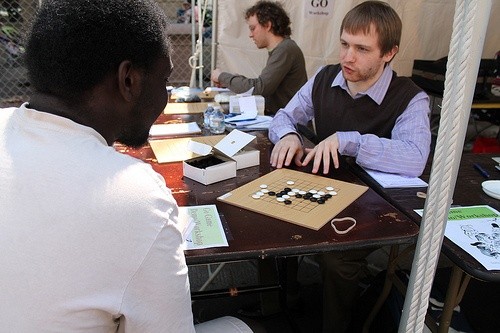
[215,129,260,170]
[182,140,237,186]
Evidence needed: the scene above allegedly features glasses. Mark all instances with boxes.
[247,13,268,33]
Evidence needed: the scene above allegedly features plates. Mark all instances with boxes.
[481,180,500,200]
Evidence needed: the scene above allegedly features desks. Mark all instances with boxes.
[113,93,500,333]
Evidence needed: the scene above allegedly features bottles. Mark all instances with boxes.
[204,104,225,134]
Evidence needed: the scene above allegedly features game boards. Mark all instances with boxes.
[170,91,219,99]
[147,135,227,163]
[217,168,369,231]
[164,102,225,115]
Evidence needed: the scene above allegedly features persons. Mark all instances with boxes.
[267,0,432,178]
[0,0,255,333]
[210,0,308,116]
